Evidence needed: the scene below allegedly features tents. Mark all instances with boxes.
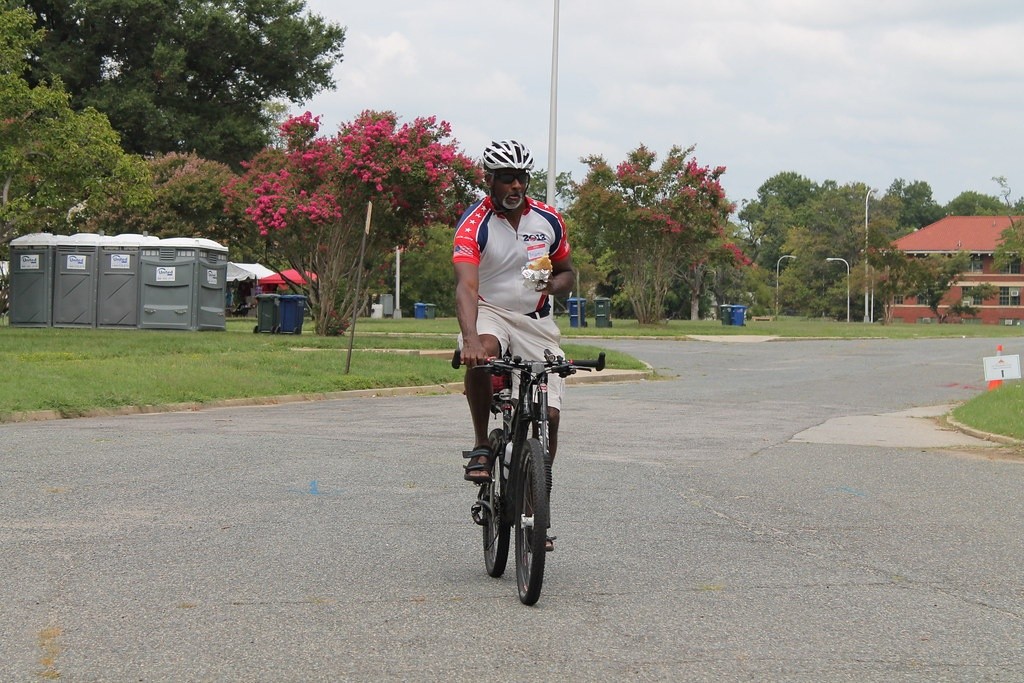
[226,261,276,282]
[257,268,318,296]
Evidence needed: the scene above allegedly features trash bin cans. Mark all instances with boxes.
[413,302,436,319]
[719,304,747,326]
[567,298,588,328]
[593,296,613,328]
[253,292,308,334]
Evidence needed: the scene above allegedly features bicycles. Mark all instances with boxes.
[451,348,607,605]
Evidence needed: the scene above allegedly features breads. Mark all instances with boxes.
[526,255,552,271]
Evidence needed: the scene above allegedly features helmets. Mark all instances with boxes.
[482,139,534,171]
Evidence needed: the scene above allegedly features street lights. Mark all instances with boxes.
[776,255,797,316]
[825,257,850,324]
[858,187,880,323]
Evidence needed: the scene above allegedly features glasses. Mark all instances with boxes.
[485,171,531,185]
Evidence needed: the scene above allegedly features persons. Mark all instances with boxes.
[452,138,578,552]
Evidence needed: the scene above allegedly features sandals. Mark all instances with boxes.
[462,444,493,482]
[545,535,556,551]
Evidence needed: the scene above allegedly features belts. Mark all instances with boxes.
[524,309,550,320]
